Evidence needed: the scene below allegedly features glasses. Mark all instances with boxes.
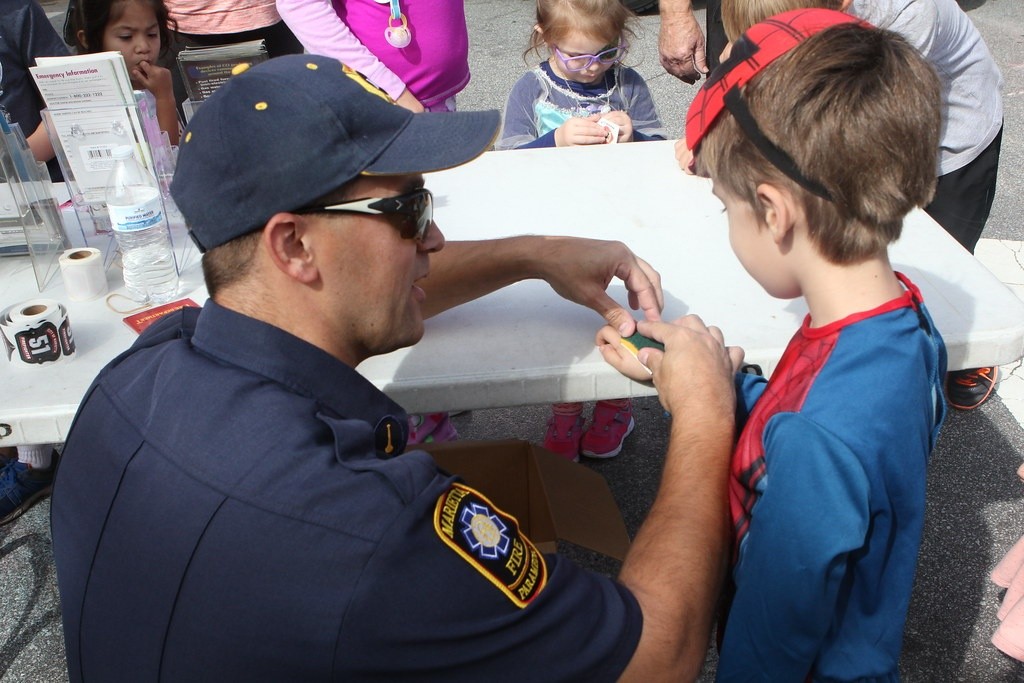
[291,187,434,242]
[551,34,629,72]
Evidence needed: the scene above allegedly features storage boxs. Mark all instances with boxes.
[418,435,632,561]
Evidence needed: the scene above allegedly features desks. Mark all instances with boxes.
[1,143,1024,451]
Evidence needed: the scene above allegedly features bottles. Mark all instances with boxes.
[103,145,179,305]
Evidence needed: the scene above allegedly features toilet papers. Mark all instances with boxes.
[57,245,109,300]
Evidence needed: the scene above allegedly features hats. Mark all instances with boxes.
[169,55,501,253]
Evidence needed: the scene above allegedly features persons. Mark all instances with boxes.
[52,53,746,683]
[596,8,947,683]
[0,0,1006,525]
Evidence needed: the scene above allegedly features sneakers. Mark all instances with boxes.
[0,448,62,524]
[543,413,585,464]
[947,365,998,411]
[580,396,635,458]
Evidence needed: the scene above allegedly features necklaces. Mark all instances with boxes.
[560,70,610,113]
